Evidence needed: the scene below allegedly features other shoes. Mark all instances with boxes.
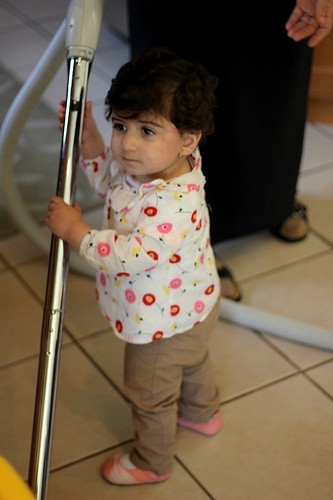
[101,454,171,485]
[179,411,221,435]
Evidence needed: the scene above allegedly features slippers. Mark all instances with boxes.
[266,204,308,243]
[214,252,241,302]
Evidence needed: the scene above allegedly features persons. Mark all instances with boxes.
[44,54,223,486]
[126,0,333,301]
